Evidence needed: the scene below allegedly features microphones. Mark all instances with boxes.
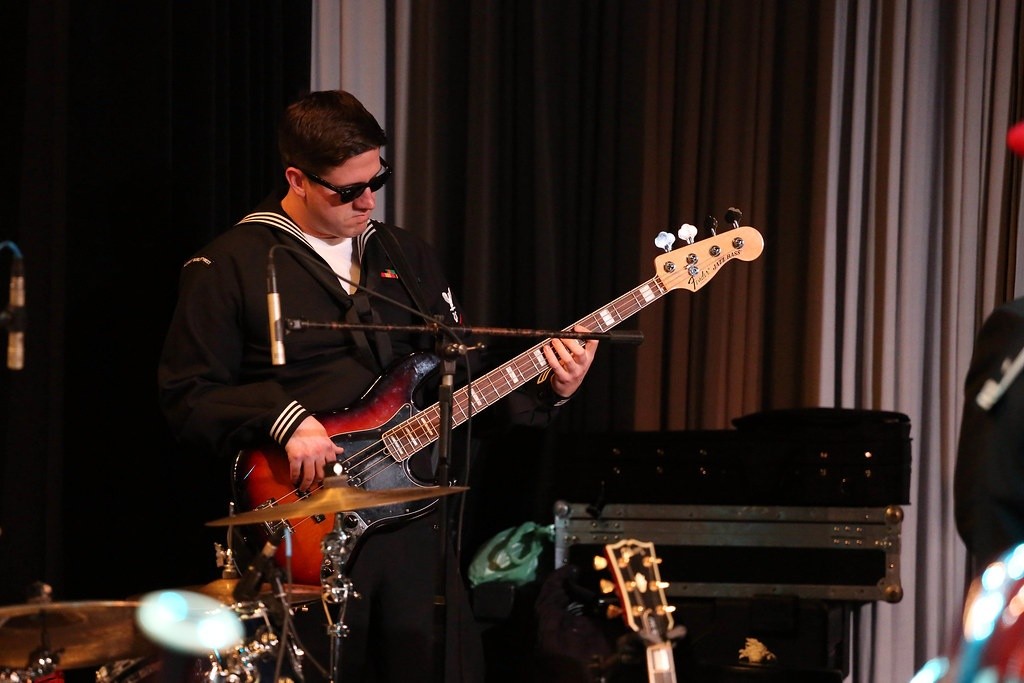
[9,256,26,369]
[267,252,286,365]
[240,526,286,597]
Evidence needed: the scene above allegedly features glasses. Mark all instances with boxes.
[285,155,393,203]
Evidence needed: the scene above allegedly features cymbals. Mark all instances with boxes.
[0,585,153,674]
[203,485,473,527]
[188,578,326,621]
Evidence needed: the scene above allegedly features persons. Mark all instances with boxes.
[156,91,599,683]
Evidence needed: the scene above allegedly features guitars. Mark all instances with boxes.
[233,207,766,598]
[591,538,687,683]
